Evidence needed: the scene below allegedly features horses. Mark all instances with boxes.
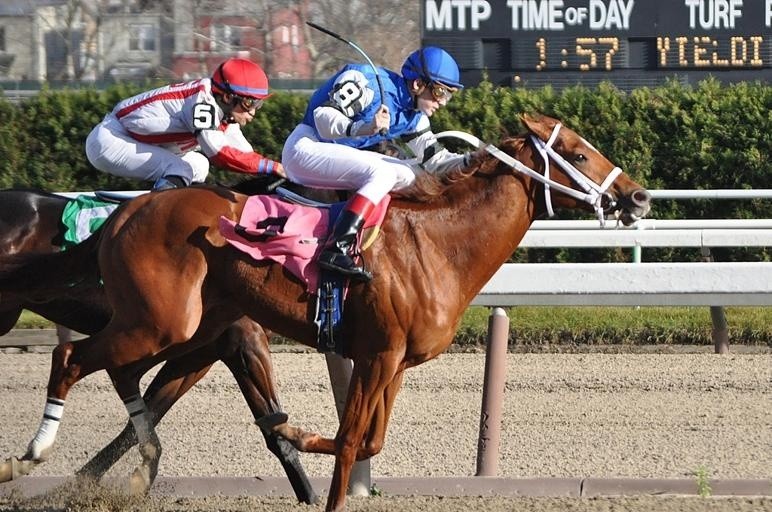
[0,111,653,512]
[0,137,400,509]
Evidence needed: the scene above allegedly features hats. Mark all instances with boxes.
[400,46,465,88]
[211,57,274,101]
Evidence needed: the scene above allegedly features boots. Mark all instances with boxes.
[317,208,374,281]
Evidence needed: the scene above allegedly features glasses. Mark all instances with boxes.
[227,95,265,111]
[425,81,454,101]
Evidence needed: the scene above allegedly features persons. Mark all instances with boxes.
[278,45,491,278]
[82,57,287,191]
